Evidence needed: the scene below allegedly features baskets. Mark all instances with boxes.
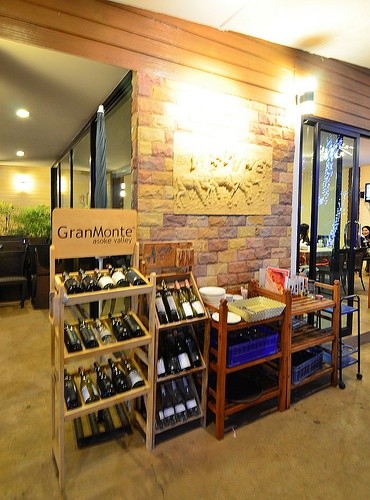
[274,347,324,384]
[209,327,279,368]
[226,296,286,324]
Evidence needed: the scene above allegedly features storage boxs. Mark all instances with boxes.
[228,295,286,322]
[197,325,278,367]
[256,346,324,384]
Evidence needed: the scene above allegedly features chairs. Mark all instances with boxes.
[0,245,50,310]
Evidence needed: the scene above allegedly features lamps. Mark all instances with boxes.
[295,77,315,115]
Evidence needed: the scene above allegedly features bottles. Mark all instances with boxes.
[65,310,144,352]
[155,279,205,324]
[143,375,202,429]
[158,328,204,377]
[62,262,147,295]
[64,356,146,411]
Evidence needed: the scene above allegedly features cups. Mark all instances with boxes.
[226,294,234,302]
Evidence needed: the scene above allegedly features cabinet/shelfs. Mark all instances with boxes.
[51,209,363,491]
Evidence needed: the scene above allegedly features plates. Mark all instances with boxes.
[199,286,226,314]
[212,310,243,323]
[233,295,243,300]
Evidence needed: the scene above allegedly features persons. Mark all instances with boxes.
[300,221,370,277]
